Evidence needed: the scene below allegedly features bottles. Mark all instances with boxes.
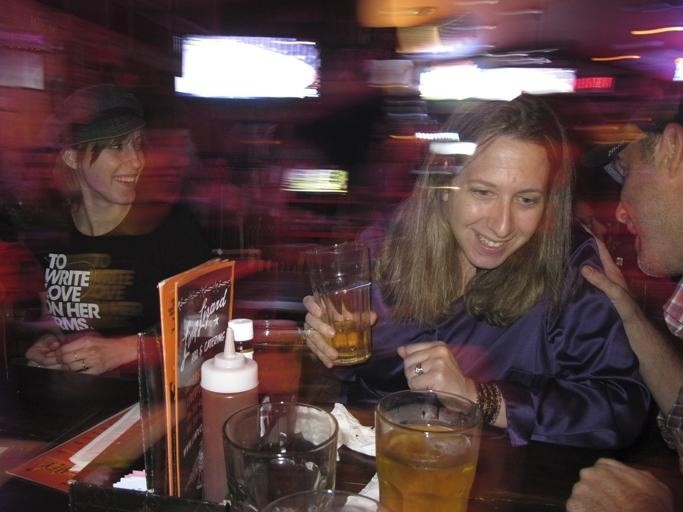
[225,317,256,362]
[198,324,264,501]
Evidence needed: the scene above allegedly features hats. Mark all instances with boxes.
[38,83,146,148]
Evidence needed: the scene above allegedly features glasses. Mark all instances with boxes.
[598,142,631,187]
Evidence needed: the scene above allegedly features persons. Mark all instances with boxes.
[25,83,212,376]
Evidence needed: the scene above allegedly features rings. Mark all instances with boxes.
[55,348,86,372]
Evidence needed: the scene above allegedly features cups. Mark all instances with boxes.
[222,399,340,512]
[262,489,395,512]
[301,241,375,369]
[371,388,484,512]
